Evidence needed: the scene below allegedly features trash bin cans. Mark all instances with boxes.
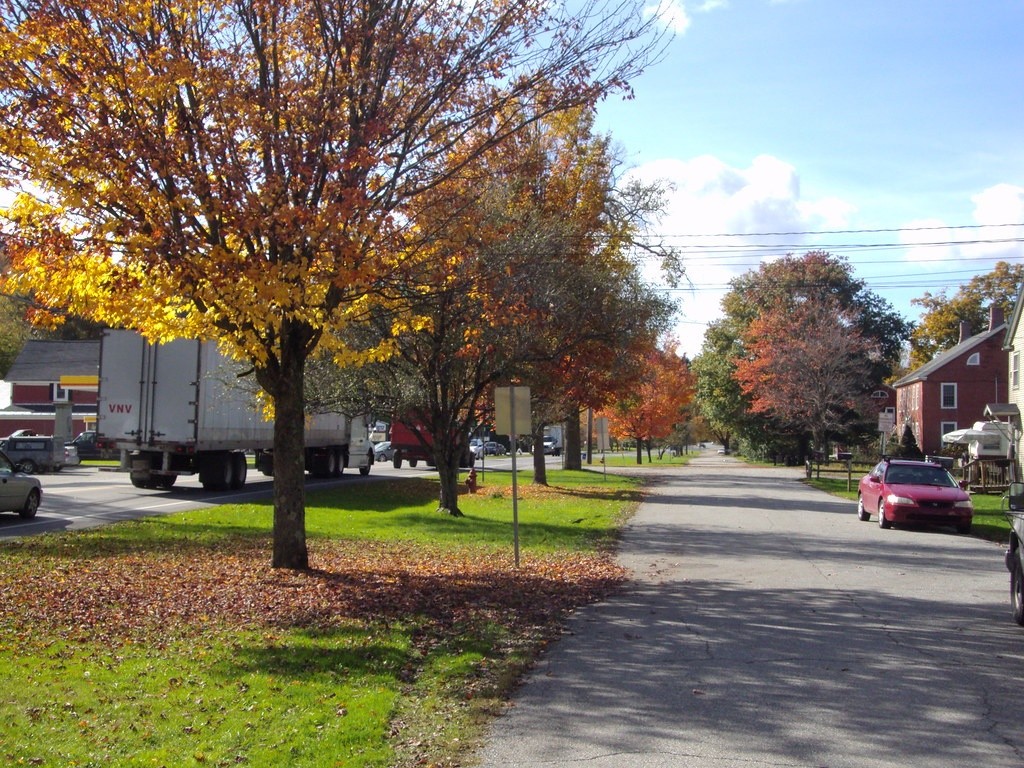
[783,455,792,466]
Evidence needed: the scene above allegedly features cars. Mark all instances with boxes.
[718,448,725,455]
[1001,480,1023,625]
[0,450,43,521]
[484,441,507,456]
[469,438,485,461]
[373,441,394,462]
[65,430,121,460]
[857,455,973,534]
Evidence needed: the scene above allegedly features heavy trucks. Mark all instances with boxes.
[94,326,376,494]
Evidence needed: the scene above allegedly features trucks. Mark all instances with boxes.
[531,425,563,456]
[391,408,476,468]
[368,420,390,443]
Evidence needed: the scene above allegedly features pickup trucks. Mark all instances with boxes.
[0,429,80,474]
